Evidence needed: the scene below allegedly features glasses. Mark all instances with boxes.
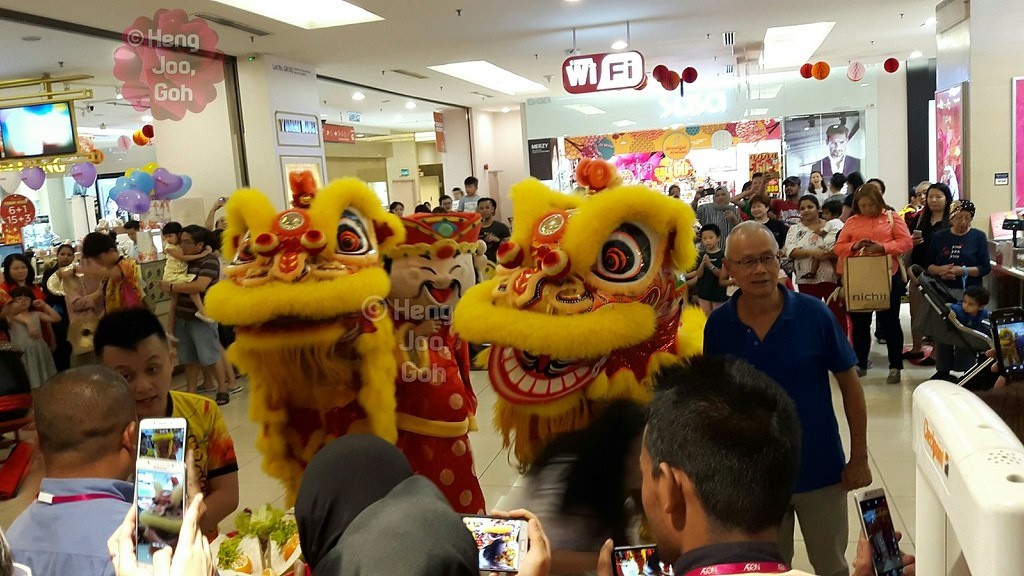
[181,240,201,246]
[728,255,778,267]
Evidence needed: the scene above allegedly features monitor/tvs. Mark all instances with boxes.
[0,101,75,159]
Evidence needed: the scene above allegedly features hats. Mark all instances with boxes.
[782,176,801,184]
[950,199,975,220]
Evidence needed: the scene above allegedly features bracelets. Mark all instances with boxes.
[170,283,174,290]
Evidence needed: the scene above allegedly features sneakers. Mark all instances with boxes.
[196,383,218,393]
[216,391,229,406]
[855,365,866,376]
[887,369,900,383]
[227,386,243,394]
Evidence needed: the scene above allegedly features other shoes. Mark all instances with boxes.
[918,357,936,366]
[903,350,924,360]
[875,332,886,344]
[166,330,180,342]
[195,311,215,323]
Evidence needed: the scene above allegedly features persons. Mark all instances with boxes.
[851,527,915,576]
[93,306,239,549]
[668,171,1024,389]
[141,432,155,456]
[483,539,506,562]
[701,219,872,576]
[0,364,549,576]
[388,175,510,352]
[0,197,243,430]
[812,123,860,185]
[598,347,820,576]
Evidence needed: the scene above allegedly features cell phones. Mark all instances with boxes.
[991,306,1024,383]
[133,417,188,565]
[853,487,903,576]
[913,230,922,239]
[610,544,675,576]
[222,197,228,203]
[458,513,528,572]
[700,188,715,197]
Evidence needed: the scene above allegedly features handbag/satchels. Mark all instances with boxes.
[844,247,892,311]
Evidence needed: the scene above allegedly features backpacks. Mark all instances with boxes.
[898,204,915,221]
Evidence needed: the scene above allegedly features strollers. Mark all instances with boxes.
[906,263,1003,391]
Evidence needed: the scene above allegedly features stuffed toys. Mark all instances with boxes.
[382,208,487,514]
[464,519,486,550]
[151,433,178,459]
[140,477,183,559]
[201,171,407,504]
[487,524,516,567]
[453,158,708,464]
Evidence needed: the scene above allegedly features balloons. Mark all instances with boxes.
[108,162,191,214]
[70,149,105,187]
[0,166,46,194]
[117,123,154,150]
